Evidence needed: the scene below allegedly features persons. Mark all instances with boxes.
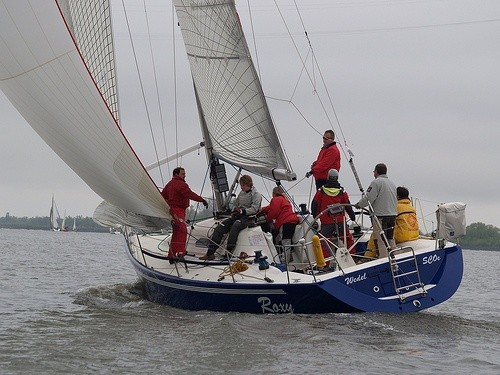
[161,167,208,262]
[257,187,300,264]
[309,130,342,219]
[198,175,262,261]
[394,186,420,245]
[311,168,357,269]
[353,163,398,262]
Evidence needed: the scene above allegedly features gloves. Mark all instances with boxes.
[202,200,208,209]
[350,215,355,221]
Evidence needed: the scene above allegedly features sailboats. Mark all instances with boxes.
[50,193,68,231]
[72,219,78,232]
[0,0,468,316]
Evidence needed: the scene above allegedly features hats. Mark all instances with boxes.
[328,169,339,176]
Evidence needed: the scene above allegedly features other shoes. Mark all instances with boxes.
[169,256,185,264]
[220,254,232,260]
[199,254,215,260]
[323,265,330,271]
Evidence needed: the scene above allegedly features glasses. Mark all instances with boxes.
[323,136,331,140]
